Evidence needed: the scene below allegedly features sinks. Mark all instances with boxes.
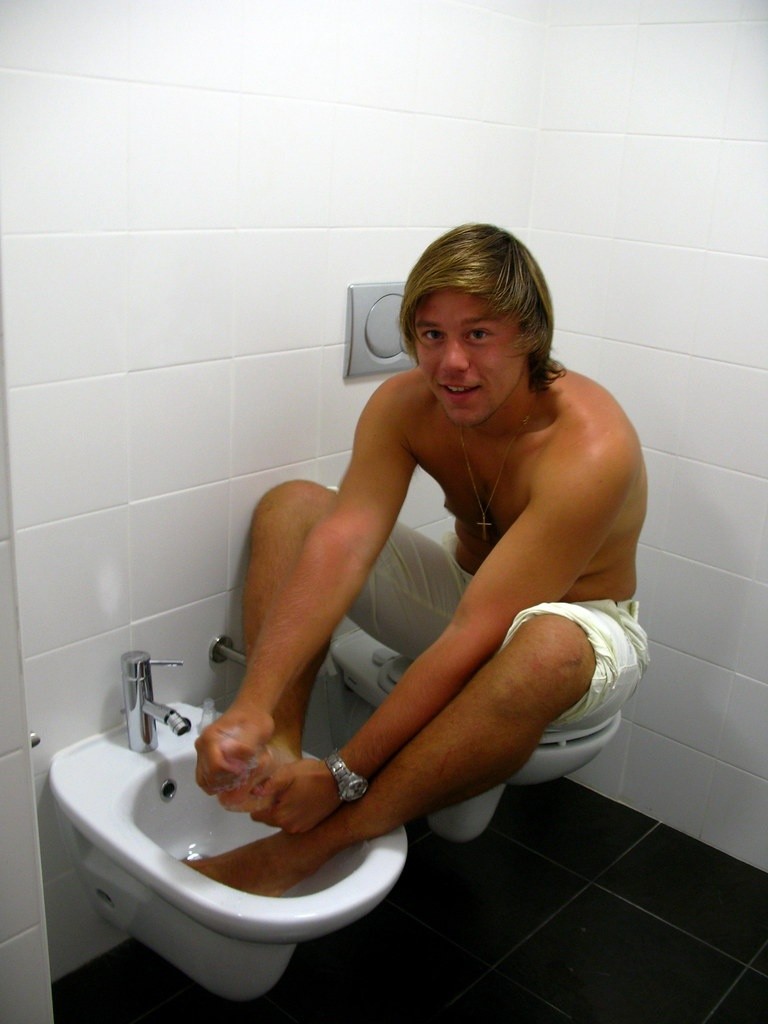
[124,743,408,946]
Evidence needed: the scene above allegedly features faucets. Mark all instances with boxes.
[119,648,191,753]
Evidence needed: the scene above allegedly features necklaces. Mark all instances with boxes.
[459,414,529,540]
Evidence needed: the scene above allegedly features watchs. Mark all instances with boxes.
[325,751,367,800]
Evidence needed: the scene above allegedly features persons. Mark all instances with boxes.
[181,223,652,897]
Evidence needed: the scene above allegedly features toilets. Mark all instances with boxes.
[328,626,628,843]
